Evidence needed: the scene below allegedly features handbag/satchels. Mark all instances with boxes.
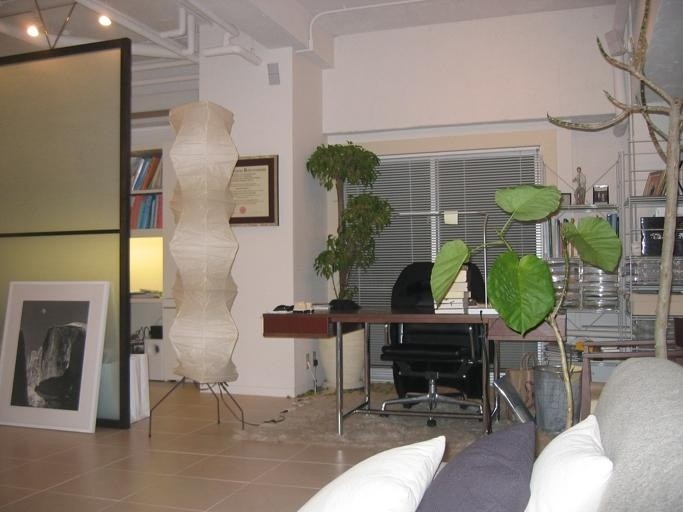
[501,352,546,424]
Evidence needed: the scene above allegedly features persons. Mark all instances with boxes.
[572,167,586,205]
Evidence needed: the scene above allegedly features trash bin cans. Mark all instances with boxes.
[533,364,583,431]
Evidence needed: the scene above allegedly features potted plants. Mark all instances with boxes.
[305,138,397,392]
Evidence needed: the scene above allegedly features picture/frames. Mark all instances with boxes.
[642,168,667,197]
[0,282,110,435]
[229,154,281,228]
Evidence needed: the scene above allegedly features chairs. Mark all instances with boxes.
[379,262,493,428]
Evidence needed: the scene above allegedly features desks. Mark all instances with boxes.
[263,311,568,436]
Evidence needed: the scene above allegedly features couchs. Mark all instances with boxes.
[432,363,683,512]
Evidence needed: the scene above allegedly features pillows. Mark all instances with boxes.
[296,433,450,512]
[415,418,538,512]
[523,413,613,511]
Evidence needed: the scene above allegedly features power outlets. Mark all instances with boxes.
[310,351,318,370]
[303,352,311,370]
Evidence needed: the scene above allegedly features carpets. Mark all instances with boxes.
[233,383,600,462]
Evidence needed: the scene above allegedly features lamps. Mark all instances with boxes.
[148,98,245,439]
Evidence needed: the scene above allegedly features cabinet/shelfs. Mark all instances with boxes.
[622,195,683,353]
[131,293,161,384]
[537,148,625,346]
[129,142,170,238]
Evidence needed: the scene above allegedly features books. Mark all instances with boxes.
[130,194,163,229]
[547,214,620,258]
[468,306,499,315]
[434,266,471,316]
[130,155,162,190]
